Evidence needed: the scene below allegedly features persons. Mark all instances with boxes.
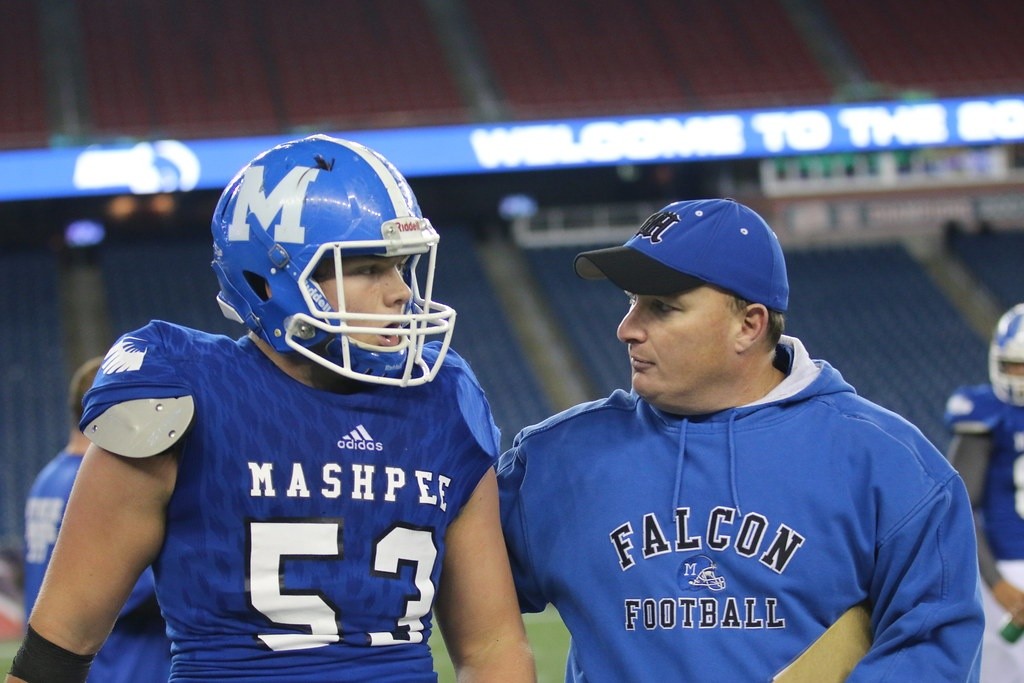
[495,199,990,683]
[940,300,1024,683]
[24,357,173,683]
[3,134,540,683]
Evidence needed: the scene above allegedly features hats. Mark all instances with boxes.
[574,197,789,313]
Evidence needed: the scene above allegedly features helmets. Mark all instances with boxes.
[211,134,456,386]
[988,303,1024,406]
